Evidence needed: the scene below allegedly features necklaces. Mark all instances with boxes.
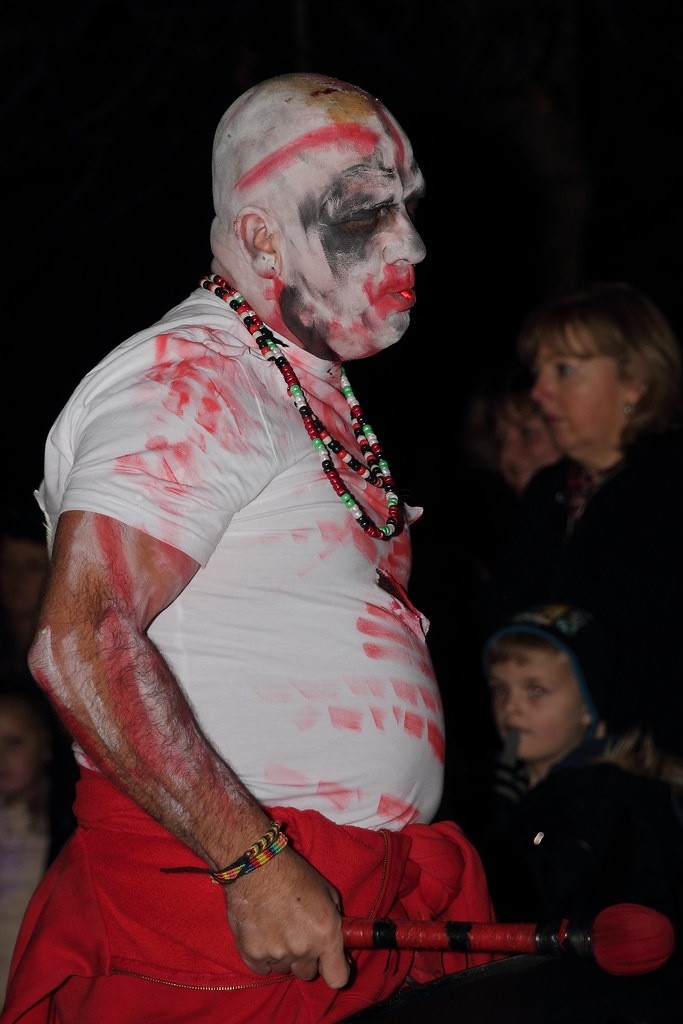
[200,272,406,540]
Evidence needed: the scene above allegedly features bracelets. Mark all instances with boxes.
[159,820,289,888]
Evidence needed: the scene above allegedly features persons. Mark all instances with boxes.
[1,70,495,1024]
[1,289,683,1023]
